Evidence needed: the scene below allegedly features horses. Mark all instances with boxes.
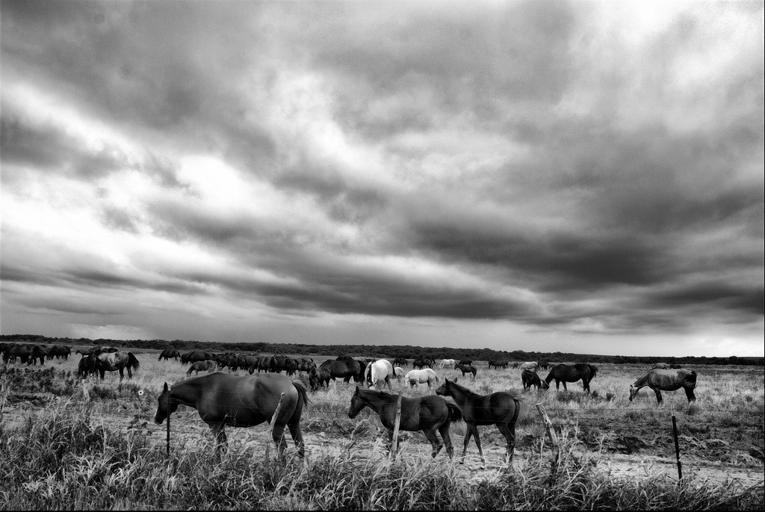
[0,342,140,382]
[487,359,553,371]
[345,383,463,465]
[542,362,600,394]
[628,360,698,406]
[520,368,542,390]
[154,345,477,393]
[151,371,315,459]
[434,376,526,466]
